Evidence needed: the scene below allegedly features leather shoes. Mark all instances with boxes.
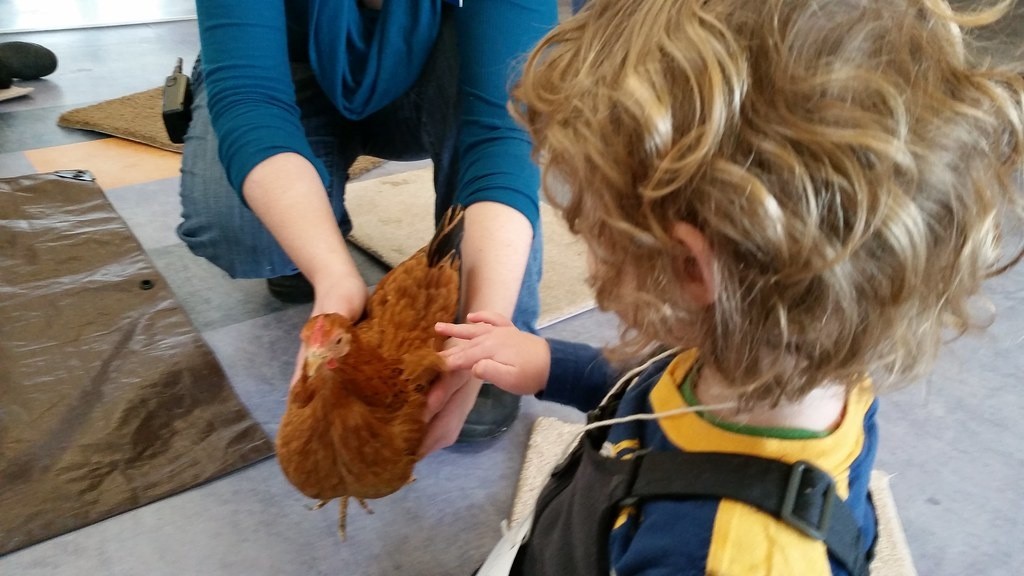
[268,271,315,304]
[458,384,520,441]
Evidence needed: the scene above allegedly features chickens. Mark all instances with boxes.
[274,200,466,543]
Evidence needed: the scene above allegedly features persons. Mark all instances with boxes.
[176,0,559,455]
[435,0,1024,576]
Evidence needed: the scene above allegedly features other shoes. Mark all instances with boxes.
[0,41,58,81]
